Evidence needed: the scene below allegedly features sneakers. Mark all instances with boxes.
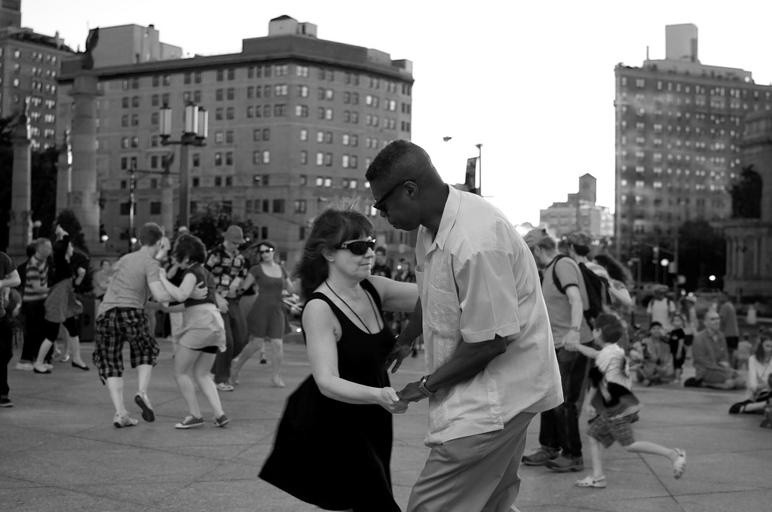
[729,400,753,414]
[174,414,205,429]
[546,454,584,471]
[272,375,285,387]
[15,349,70,374]
[113,411,138,428]
[670,448,686,479]
[574,475,606,488]
[213,416,228,427]
[0,395,14,407]
[210,369,239,392]
[641,378,706,388]
[523,444,559,465]
[135,392,155,422]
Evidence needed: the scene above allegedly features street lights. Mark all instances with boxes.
[161,103,209,233]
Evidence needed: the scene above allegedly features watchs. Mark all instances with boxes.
[417,375,434,400]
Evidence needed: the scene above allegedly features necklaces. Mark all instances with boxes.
[325,280,385,335]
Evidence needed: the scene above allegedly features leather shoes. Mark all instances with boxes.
[72,359,89,371]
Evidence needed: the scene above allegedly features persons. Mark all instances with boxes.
[93,223,209,427]
[556,313,687,487]
[258,209,418,512]
[728,330,772,429]
[373,245,392,276]
[523,229,594,473]
[229,242,298,389]
[393,261,416,345]
[0,224,109,407]
[568,234,751,390]
[204,225,251,391]
[366,139,564,511]
[159,234,229,429]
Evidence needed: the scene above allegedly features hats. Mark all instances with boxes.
[567,231,591,246]
[220,225,247,244]
[524,227,549,248]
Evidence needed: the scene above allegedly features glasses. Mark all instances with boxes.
[372,179,416,212]
[259,248,273,254]
[336,239,377,255]
